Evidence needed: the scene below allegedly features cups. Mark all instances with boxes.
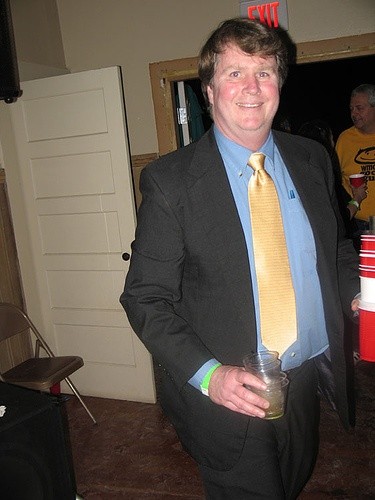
[349,174,365,189]
[356,234,375,362]
[243,350,289,420]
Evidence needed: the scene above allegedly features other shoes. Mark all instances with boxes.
[346,350,360,366]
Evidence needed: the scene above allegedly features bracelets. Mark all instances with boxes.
[201,363,223,396]
[352,201,359,209]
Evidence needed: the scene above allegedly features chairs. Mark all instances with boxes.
[0,302,98,426]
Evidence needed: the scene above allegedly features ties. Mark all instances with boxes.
[248,153,297,360]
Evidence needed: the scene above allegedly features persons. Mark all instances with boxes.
[120,18,360,500]
[335,84,375,254]
[298,119,367,244]
